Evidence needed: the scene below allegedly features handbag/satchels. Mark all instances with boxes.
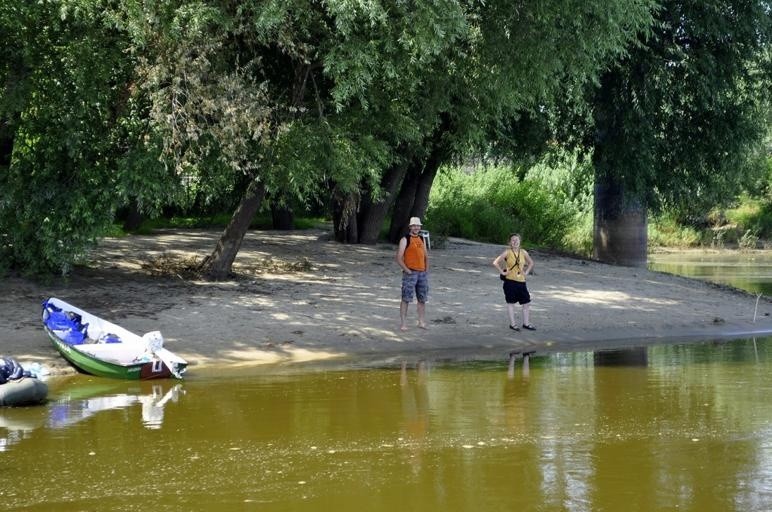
[500,269,507,281]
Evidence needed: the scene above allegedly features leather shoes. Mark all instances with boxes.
[510,324,536,331]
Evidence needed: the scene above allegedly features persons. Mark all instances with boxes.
[503,350,531,440]
[399,359,428,480]
[396,217,431,331]
[492,234,537,332]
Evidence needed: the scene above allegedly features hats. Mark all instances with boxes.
[408,216,423,226]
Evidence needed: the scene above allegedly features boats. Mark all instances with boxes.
[0,407,46,456]
[48,379,184,432]
[41,295,192,380]
[0,354,51,407]
